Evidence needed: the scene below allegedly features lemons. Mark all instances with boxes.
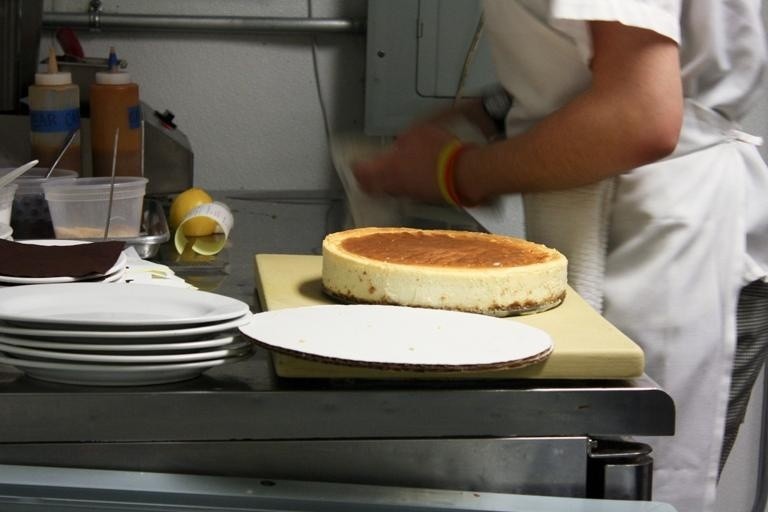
[169,187,216,237]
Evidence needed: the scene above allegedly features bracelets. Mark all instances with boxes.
[438,141,478,211]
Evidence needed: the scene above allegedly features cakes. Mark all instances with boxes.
[321,226,569,318]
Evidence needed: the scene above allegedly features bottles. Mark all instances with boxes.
[29,45,143,177]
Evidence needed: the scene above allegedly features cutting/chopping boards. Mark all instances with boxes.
[255,254,646,379]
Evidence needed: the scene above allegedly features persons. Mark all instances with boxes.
[350,1,766,512]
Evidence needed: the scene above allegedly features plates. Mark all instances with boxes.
[0,240,256,388]
